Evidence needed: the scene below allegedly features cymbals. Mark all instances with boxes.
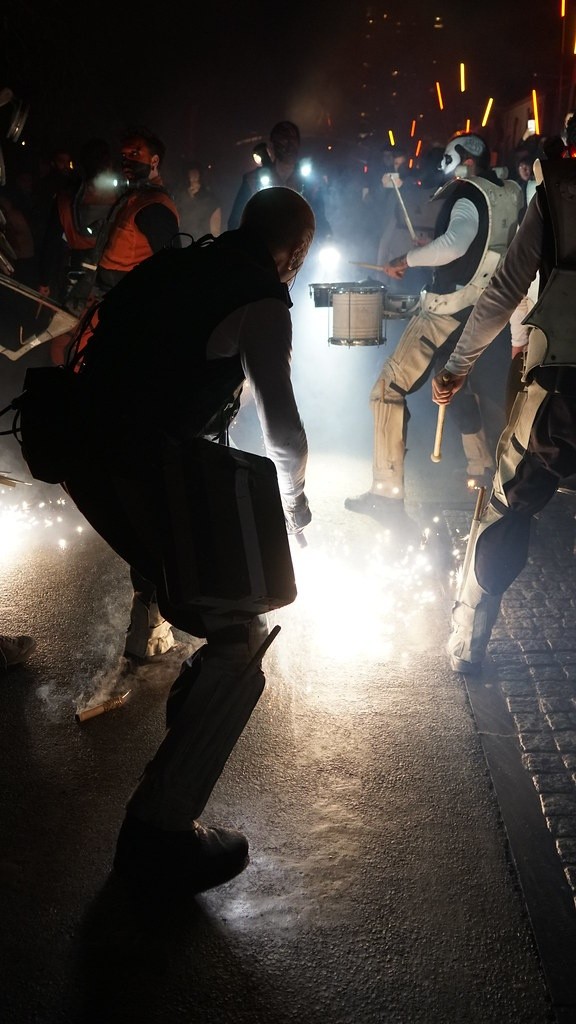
[358,276,387,288]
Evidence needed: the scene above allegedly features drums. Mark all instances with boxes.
[310,281,356,308]
[328,285,388,348]
[385,291,420,320]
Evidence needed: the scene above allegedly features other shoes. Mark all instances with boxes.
[0,635,37,673]
[108,819,249,908]
[450,467,496,489]
[448,655,483,679]
[344,492,404,521]
[123,640,195,663]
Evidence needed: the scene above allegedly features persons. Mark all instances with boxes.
[0,121,576,673]
[59,185,317,900]
[0,634,38,669]
